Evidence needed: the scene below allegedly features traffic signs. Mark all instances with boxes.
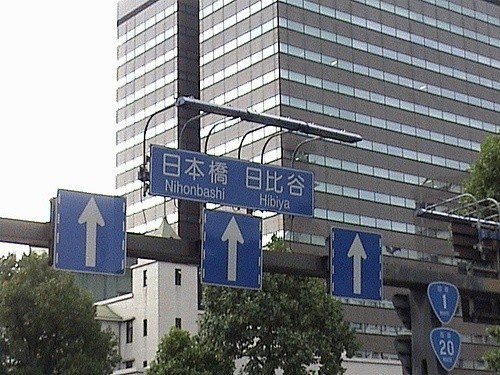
[429,328,462,372]
[149,145,315,291]
